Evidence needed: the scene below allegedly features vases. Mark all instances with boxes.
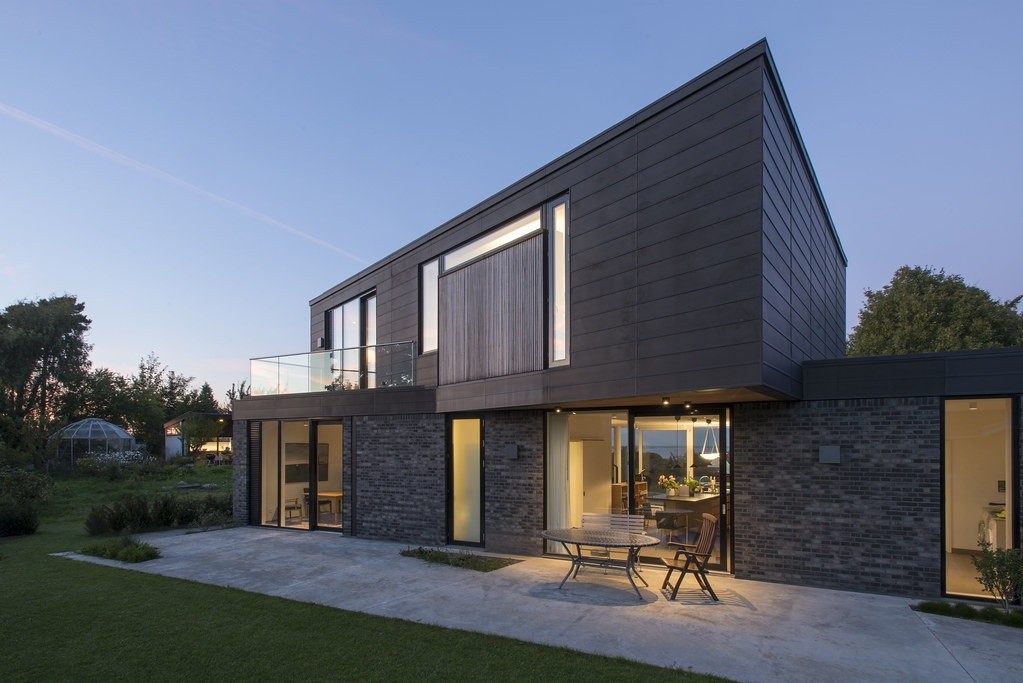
[665,487,675,497]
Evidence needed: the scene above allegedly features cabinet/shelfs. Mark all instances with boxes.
[612,481,648,516]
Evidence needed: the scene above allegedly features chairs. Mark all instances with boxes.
[304,487,310,516]
[661,512,720,601]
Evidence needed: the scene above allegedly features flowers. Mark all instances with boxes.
[657,474,679,489]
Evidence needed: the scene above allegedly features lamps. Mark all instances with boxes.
[700,418,720,460]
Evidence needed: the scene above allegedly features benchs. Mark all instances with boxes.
[570,513,646,575]
[285,497,302,518]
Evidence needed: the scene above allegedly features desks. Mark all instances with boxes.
[301,491,343,514]
[541,528,660,600]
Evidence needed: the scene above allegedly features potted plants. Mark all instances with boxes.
[684,475,698,497]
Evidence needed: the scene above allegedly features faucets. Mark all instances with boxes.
[698,475,710,495]
